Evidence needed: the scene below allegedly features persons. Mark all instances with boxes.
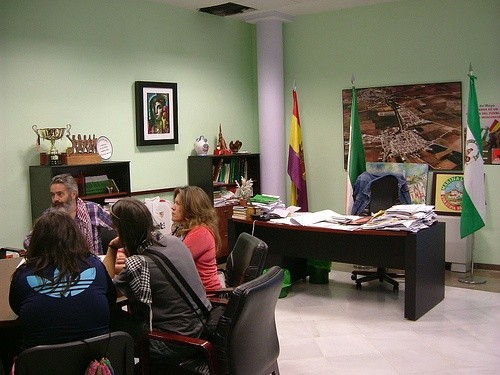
[102,199,212,359]
[24,174,114,254]
[170,185,229,308]
[9,211,119,357]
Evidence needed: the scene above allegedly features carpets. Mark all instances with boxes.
[275,269,500,375]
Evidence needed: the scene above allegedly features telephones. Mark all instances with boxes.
[251,212,281,221]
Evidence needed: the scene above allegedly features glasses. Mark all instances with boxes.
[109,200,121,221]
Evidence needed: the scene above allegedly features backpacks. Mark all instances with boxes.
[79,331,115,375]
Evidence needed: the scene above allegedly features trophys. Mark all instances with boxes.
[32,124,71,165]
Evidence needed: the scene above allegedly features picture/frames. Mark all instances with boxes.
[431,170,486,217]
[135,81,180,147]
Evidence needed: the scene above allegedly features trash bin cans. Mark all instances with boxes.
[309,257,331,285]
[264,269,289,299]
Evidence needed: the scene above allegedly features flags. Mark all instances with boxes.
[345,85,367,215]
[459,73,487,239]
[287,89,308,213]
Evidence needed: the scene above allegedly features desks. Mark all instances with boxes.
[0,255,127,375]
[224,214,447,322]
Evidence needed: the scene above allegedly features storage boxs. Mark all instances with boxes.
[38,152,101,165]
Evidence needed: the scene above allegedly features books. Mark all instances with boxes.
[212,157,282,220]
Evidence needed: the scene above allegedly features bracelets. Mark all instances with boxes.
[108,244,118,251]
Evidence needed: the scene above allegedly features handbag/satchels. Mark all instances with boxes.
[197,305,232,365]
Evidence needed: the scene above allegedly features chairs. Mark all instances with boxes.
[100,224,117,254]
[8,330,134,375]
[205,231,269,295]
[351,171,411,296]
[136,265,286,375]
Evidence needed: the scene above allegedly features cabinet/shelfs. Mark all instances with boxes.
[29,161,132,227]
[187,154,260,261]
